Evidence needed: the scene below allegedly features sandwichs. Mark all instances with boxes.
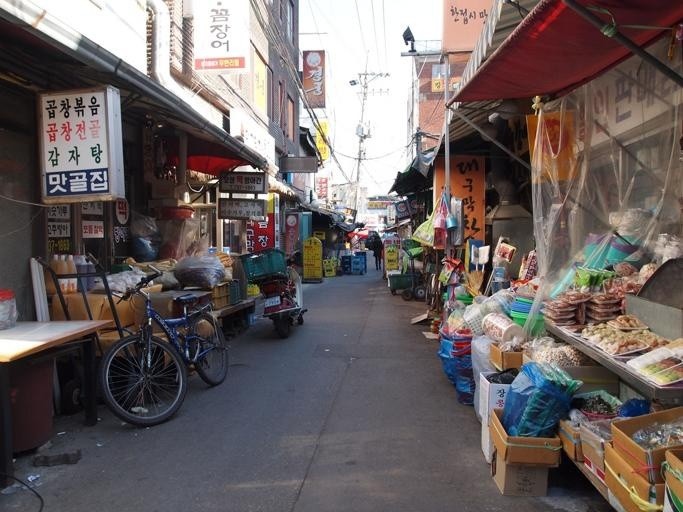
[466,271,484,297]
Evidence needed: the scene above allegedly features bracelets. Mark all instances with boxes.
[510,294,543,335]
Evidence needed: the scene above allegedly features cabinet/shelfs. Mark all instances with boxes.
[48,254,94,294]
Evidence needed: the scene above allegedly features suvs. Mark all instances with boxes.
[508,297,549,336]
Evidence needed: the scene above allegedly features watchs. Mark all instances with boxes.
[239,245,308,341]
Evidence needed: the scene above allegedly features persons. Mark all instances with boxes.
[373,235,382,270]
